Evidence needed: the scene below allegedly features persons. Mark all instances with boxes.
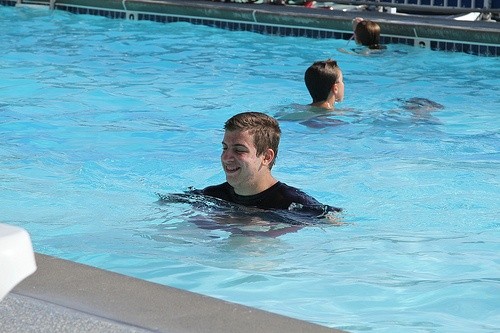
[183,112,335,221]
[159,216,316,269]
[350,17,387,52]
[304,59,344,113]
[358,96,444,137]
